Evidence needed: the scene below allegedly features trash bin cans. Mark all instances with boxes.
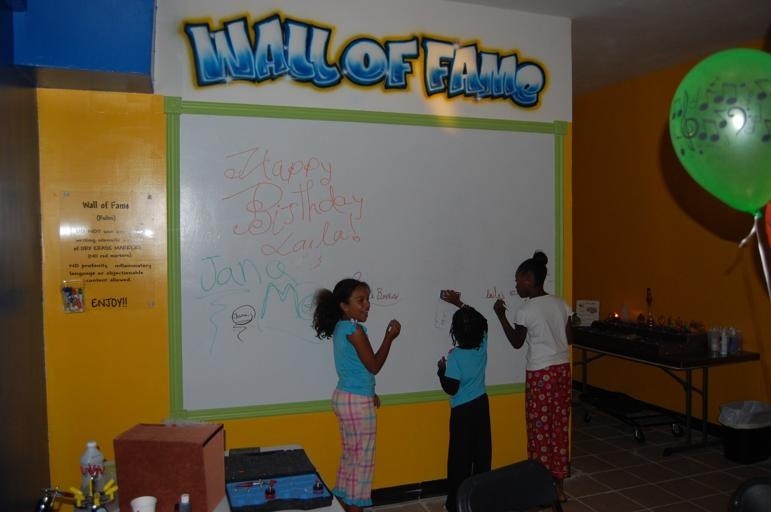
[719,401,771,465]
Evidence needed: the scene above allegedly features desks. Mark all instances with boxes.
[572,324,761,456]
[207,445,346,512]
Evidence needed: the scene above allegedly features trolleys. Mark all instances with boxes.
[577,384,687,444]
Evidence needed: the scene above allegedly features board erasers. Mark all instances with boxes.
[441,290,461,302]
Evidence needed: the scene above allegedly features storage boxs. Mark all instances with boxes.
[114,422,226,512]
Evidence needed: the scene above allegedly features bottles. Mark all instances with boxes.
[179,494,191,512]
[81,442,105,490]
[706,325,739,355]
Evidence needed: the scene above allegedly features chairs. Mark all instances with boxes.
[729,478,771,512]
[454,458,564,512]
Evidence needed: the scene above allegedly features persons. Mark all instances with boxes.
[314,279,401,512]
[438,290,491,512]
[493,252,580,502]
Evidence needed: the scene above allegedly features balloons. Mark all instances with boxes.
[669,48,771,218]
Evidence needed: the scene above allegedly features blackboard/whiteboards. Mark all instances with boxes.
[163,95,565,424]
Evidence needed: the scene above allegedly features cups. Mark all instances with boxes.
[130,496,157,512]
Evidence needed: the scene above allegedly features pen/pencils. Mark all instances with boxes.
[502,304,510,310]
[388,325,392,332]
